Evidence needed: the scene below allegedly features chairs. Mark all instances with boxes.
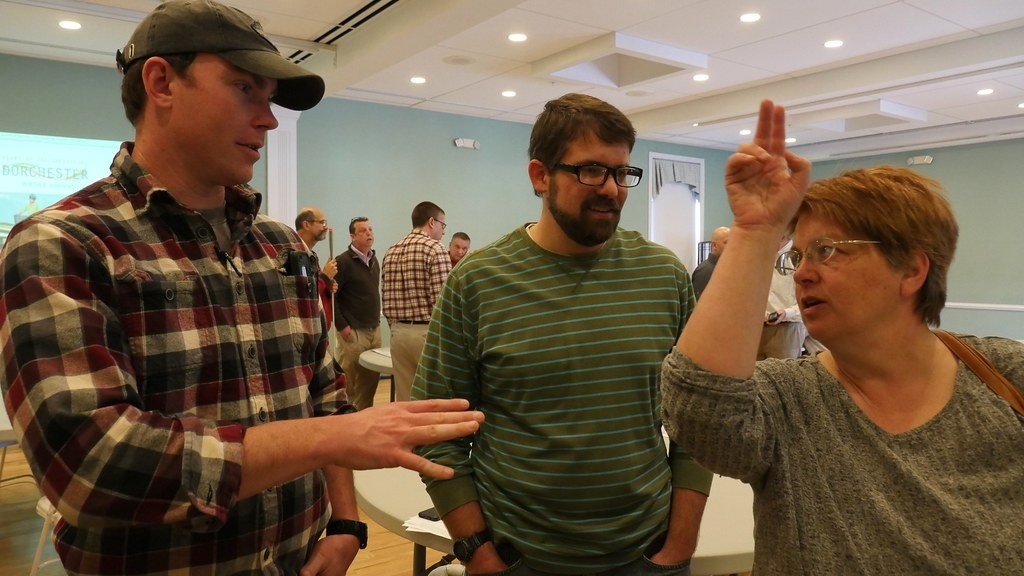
[29,495,63,576]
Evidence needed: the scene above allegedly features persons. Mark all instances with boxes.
[0,0,487,576]
[756,237,809,362]
[17,193,39,218]
[691,225,731,304]
[406,90,718,576]
[658,97,1022,576]
[333,216,381,411]
[447,232,472,269]
[294,206,338,334]
[380,200,453,405]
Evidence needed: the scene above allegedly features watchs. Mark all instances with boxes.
[326,519,368,549]
[451,527,494,565]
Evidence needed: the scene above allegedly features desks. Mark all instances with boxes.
[358,346,395,406]
[350,430,756,576]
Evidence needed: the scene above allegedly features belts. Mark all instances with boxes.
[400,319,431,324]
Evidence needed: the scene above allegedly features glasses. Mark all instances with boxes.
[350,217,368,235]
[305,220,327,227]
[428,217,447,229]
[775,237,883,277]
[554,162,643,188]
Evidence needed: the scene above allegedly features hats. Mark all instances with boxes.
[115,0,325,111]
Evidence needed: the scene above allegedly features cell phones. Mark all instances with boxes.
[768,312,778,322]
